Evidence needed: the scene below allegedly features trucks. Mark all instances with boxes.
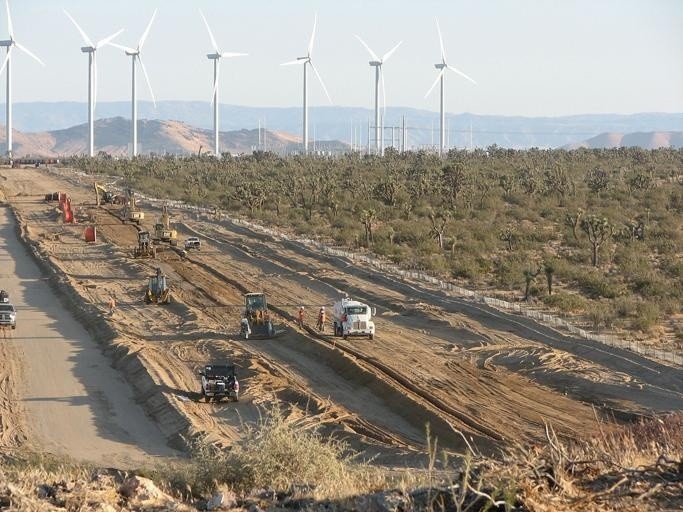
[333,297,376,340]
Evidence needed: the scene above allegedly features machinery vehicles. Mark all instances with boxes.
[241,293,273,340]
[144,267,175,305]
[94,182,177,258]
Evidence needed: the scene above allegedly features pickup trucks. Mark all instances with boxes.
[184,237,201,251]
[199,364,239,403]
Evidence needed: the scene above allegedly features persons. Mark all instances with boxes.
[107,296,117,316]
[297,306,305,330]
[316,305,325,330]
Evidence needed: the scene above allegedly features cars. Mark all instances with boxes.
[0,302,17,329]
